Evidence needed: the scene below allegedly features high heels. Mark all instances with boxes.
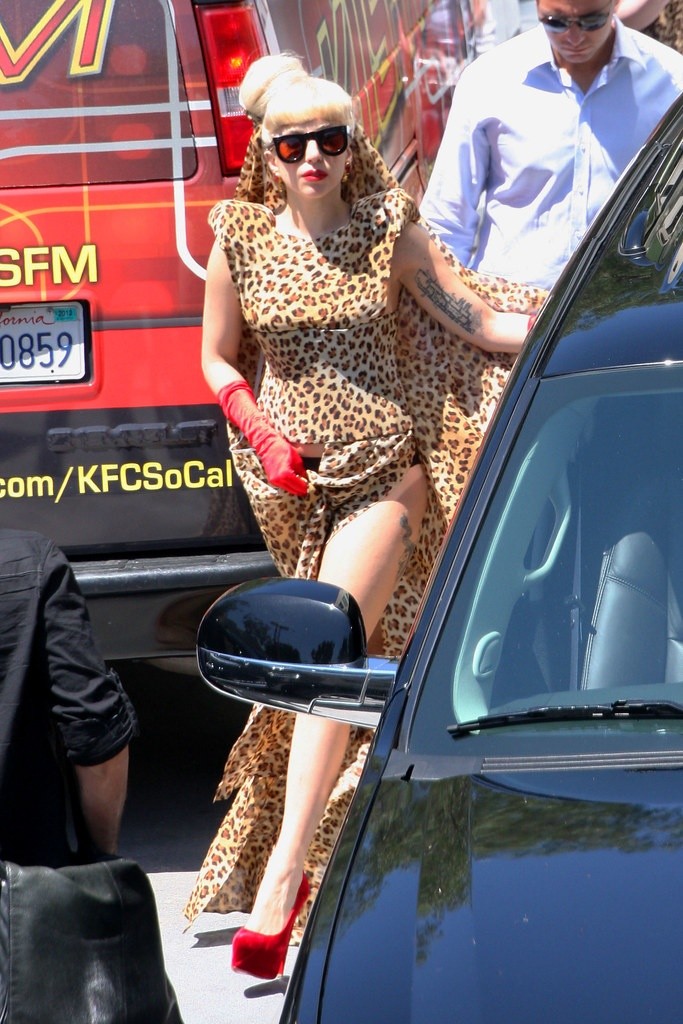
[232,869,311,980]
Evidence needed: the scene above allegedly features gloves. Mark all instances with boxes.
[216,380,308,497]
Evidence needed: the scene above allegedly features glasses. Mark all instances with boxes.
[273,126,348,163]
[538,0,616,33]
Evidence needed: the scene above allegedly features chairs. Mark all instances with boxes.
[580,436,683,691]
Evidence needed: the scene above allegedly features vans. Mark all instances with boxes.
[199,93,683,1024]
[0,0,527,746]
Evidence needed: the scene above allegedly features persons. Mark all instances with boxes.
[420,0,683,293]
[0,529,135,867]
[616,2,682,52]
[183,52,552,976]
